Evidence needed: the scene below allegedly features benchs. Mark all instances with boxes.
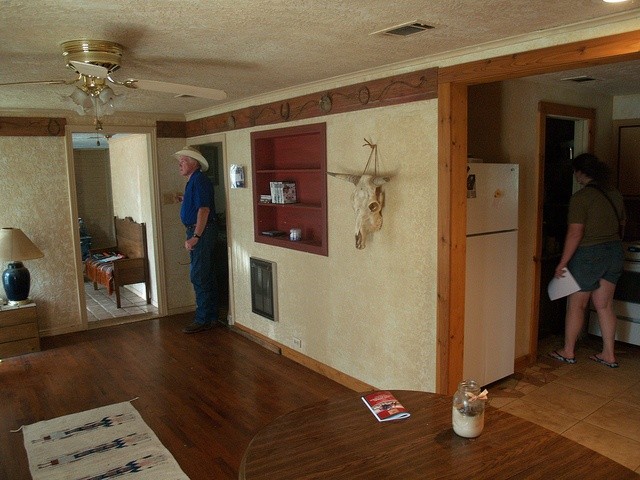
[91,216,151,309]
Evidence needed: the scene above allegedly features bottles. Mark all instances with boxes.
[290,228,302,241]
[452,381,485,438]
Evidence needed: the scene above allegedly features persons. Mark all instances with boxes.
[548,153,628,368]
[172,144,217,334]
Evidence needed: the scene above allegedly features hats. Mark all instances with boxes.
[169,146,209,173]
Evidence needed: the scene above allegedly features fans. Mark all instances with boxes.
[0,40,227,102]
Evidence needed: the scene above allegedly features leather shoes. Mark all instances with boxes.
[182,322,214,334]
[192,318,218,324]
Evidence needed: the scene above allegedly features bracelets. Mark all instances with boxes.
[193,233,201,240]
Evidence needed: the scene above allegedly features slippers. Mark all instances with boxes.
[589,354,619,368]
[547,350,577,364]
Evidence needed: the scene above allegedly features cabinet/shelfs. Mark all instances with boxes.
[0,301,42,359]
[250,122,328,256]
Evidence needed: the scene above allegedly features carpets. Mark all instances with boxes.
[10,394,190,480]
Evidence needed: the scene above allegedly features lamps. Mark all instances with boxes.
[0,228,45,306]
[49,78,139,147]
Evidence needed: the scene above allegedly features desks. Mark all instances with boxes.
[238,389,640,480]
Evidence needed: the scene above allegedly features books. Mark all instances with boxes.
[260,181,298,205]
[360,390,411,422]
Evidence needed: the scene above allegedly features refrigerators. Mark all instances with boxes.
[462,163,520,393]
